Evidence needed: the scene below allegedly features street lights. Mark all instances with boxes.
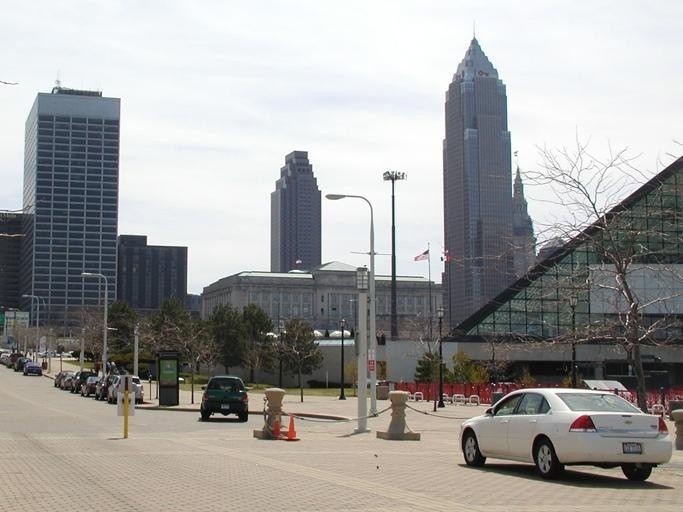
[133,325,140,377]
[568,291,581,387]
[276,316,286,388]
[383,167,409,340]
[336,316,347,400]
[349,263,372,436]
[0,293,40,355]
[325,190,380,421]
[81,272,110,378]
[434,308,447,407]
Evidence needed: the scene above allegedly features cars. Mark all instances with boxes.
[0,347,145,405]
[457,386,674,484]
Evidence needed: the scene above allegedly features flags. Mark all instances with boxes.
[440,255,448,262]
[414,250,428,261]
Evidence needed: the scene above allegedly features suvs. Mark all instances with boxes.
[199,375,248,422]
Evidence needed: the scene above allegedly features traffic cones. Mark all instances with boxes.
[285,414,300,443]
[270,412,283,440]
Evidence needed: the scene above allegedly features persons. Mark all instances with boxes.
[613,388,619,394]
[105,359,110,375]
[111,359,115,367]
[658,386,665,405]
[115,359,120,369]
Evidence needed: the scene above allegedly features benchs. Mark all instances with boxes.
[441,393,480,408]
[630,403,665,420]
[407,392,423,402]
